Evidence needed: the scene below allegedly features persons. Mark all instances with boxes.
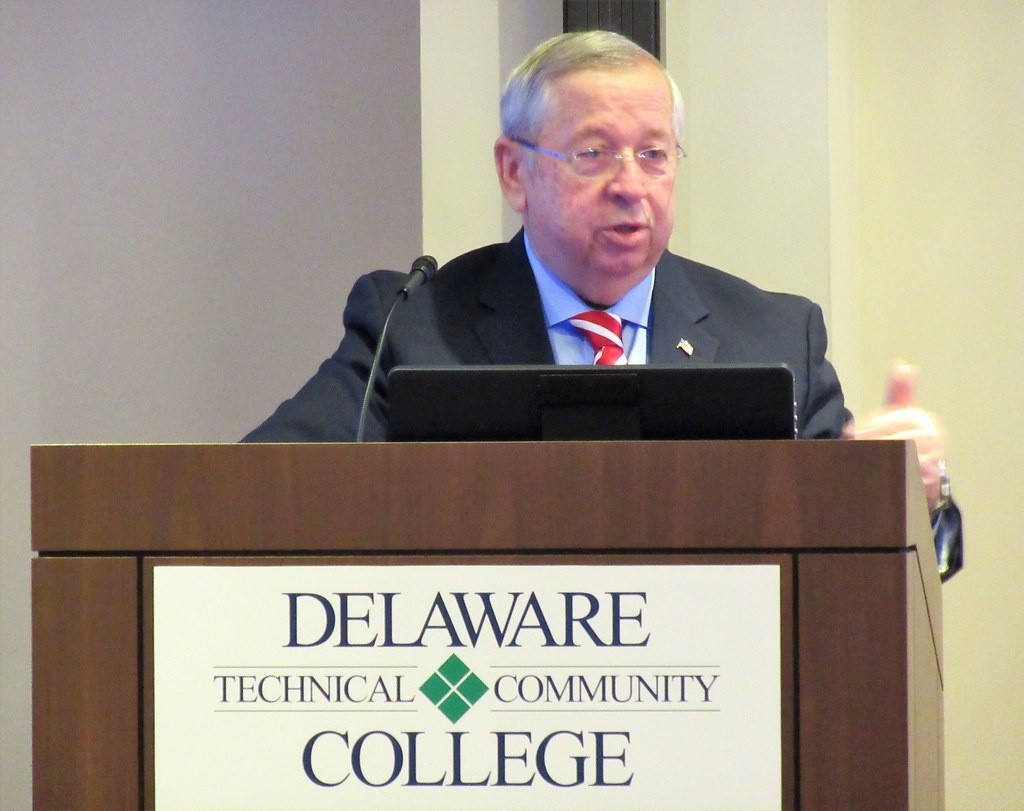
[235,31,965,583]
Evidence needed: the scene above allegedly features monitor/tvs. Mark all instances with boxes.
[385,362,798,443]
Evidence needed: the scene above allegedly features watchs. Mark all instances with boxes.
[927,459,951,517]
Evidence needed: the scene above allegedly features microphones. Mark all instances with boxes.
[398,255,437,301]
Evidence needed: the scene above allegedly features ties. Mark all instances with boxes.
[568,310,626,364]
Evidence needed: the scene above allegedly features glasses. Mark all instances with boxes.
[509,134,687,177]
